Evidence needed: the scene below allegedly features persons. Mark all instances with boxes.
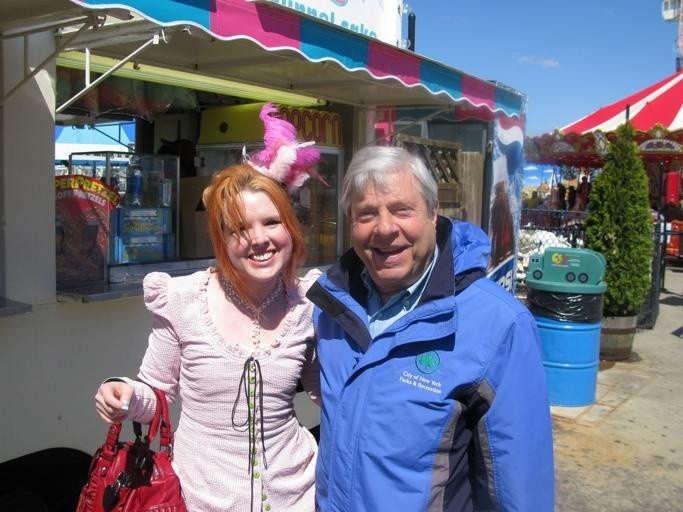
[522,171,683,236]
[92,160,322,511]
[303,143,557,512]
[491,180,515,267]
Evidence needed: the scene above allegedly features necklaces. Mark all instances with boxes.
[220,272,283,350]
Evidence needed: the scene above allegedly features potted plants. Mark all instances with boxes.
[580,121,656,362]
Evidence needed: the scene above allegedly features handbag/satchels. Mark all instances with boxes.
[75,379,189,512]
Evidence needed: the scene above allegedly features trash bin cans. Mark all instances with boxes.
[525,247,608,408]
